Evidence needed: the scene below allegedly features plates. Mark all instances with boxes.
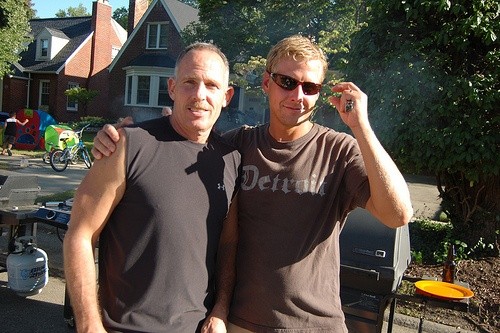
[415,280,475,300]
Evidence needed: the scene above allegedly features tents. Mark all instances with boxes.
[0,109,57,152]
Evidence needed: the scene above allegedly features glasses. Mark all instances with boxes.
[267,72,322,96]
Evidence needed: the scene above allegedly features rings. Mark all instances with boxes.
[347,88,354,93]
[346,99,354,111]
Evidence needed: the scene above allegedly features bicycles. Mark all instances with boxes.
[43,124,92,172]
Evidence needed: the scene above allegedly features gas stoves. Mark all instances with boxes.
[34,200,73,225]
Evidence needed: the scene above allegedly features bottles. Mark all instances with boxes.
[442,245,455,284]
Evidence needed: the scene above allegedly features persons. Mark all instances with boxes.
[159,106,173,117]
[91,36,414,333]
[63,44,242,333]
[0,111,29,157]
